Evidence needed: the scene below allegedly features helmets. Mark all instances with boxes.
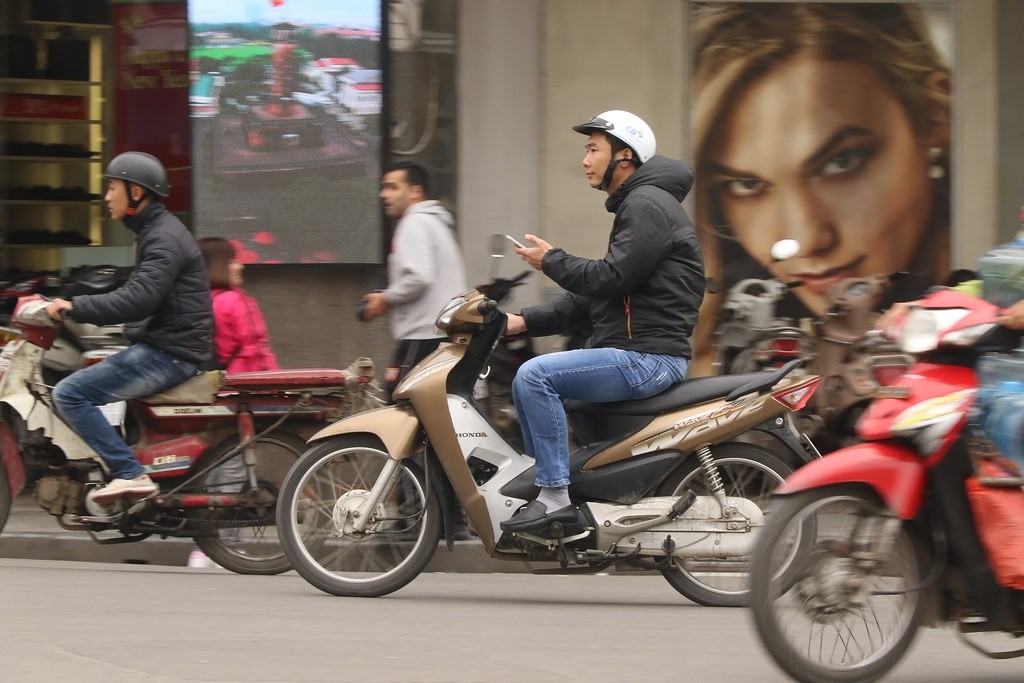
[101,152,169,197]
[572,110,656,165]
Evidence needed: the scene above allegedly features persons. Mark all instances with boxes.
[46,150,213,504]
[956,234,1024,327]
[499,110,706,535]
[361,162,474,540]
[690,0,953,380]
[187,235,280,570]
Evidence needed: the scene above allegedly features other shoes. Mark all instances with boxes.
[189,549,223,570]
[92,474,157,504]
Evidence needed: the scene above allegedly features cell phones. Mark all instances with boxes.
[505,234,525,248]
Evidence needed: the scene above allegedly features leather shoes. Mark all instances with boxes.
[499,499,580,532]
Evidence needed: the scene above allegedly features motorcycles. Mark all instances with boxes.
[275,272,826,608]
[0,265,383,575]
[749,270,1023,683]
[705,239,907,459]
